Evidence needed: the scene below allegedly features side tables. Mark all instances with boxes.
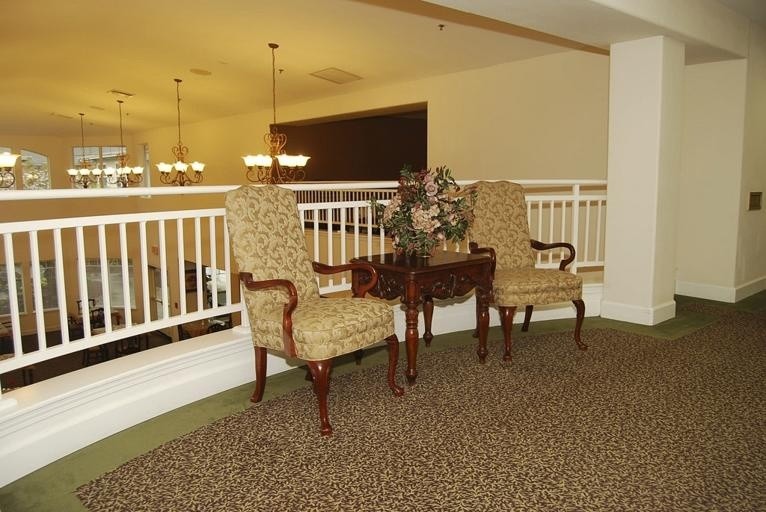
[349,249,491,386]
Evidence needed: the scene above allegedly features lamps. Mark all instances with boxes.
[1,152,21,187]
[102,100,144,186]
[240,44,310,184]
[65,114,102,187]
[155,78,206,187]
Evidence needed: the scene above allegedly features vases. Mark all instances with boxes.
[421,240,430,257]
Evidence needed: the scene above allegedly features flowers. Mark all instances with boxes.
[368,163,479,257]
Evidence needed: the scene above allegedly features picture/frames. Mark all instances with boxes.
[185,269,204,293]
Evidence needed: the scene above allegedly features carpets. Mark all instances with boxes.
[71,294,766,511]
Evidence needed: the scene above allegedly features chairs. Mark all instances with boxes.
[225,183,404,437]
[0,299,151,394]
[463,180,588,361]
